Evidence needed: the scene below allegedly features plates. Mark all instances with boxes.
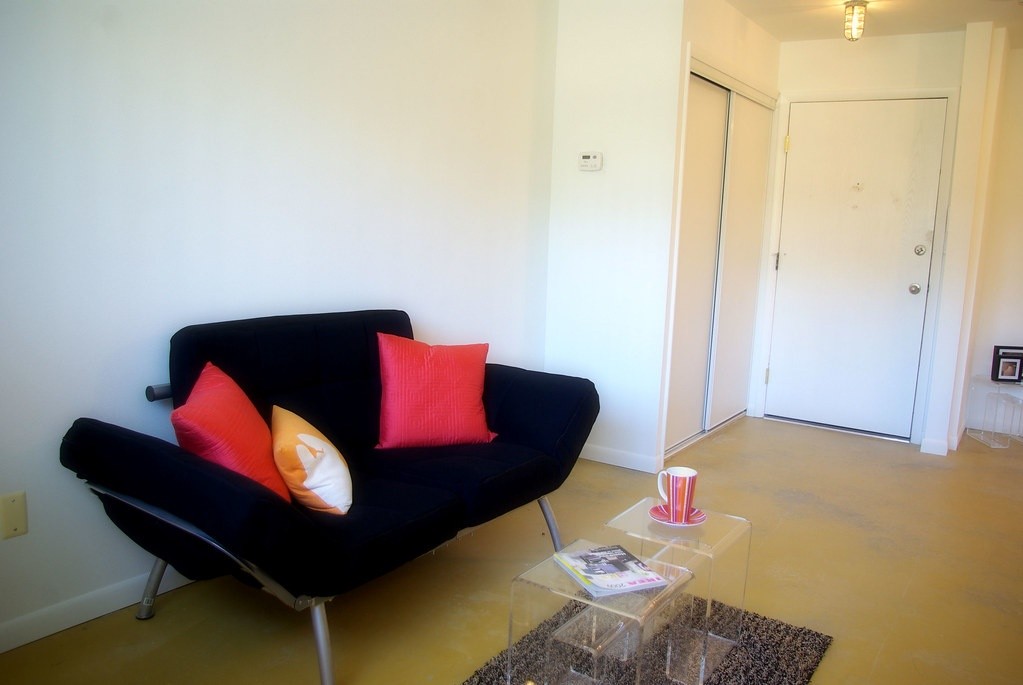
[647,504,707,527]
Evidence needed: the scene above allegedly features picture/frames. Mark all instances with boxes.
[993,345,1023,379]
[991,354,1023,383]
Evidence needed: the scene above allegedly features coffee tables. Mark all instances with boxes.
[967,375,1023,448]
[508,497,752,685]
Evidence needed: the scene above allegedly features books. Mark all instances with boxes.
[553,543,669,599]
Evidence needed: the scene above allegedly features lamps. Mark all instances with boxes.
[843,0,868,42]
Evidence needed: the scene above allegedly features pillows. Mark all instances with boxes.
[272,404,352,514]
[171,362,292,504]
[373,333,497,448]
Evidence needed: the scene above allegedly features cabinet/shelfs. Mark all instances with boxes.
[660,73,772,453]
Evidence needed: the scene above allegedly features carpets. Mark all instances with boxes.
[459,587,831,685]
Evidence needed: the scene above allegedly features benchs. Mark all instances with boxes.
[59,309,600,685]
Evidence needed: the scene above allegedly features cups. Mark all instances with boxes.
[657,466,698,524]
